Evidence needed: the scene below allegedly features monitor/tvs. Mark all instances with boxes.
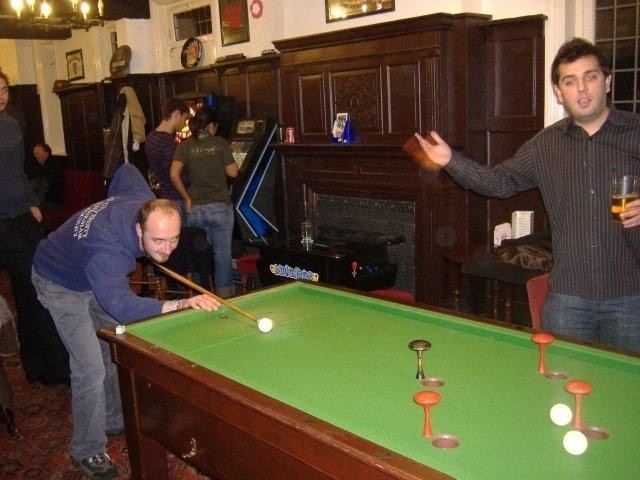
[227,150,248,170]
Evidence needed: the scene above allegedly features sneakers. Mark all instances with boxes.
[105,426,126,436]
[67,449,118,480]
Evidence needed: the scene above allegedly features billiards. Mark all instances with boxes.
[258,319,273,332]
[551,403,572,424]
[563,430,588,454]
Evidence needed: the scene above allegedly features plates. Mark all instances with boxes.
[181,37,201,68]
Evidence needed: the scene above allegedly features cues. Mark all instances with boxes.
[148,260,260,321]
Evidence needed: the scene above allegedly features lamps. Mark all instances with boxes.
[6,0,110,35]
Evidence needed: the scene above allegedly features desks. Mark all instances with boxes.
[91,274,640,480]
[439,238,552,321]
[238,229,381,288]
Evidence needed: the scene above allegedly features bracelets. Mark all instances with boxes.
[169,298,185,311]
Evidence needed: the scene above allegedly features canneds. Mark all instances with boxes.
[285,127,295,144]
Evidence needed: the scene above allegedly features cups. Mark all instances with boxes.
[610,174,639,224]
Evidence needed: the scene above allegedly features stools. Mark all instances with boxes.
[367,288,416,309]
[233,250,263,292]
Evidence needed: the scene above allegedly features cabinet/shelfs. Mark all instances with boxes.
[6,82,45,182]
[53,80,116,176]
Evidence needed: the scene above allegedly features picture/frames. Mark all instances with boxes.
[64,46,85,81]
[325,0,395,23]
[219,0,250,48]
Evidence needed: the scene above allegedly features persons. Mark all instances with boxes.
[170,107,241,296]
[1,75,68,385]
[27,143,65,215]
[29,165,222,478]
[413,37,640,354]
[145,98,212,298]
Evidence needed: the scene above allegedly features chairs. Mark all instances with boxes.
[523,272,552,330]
[38,171,96,233]
